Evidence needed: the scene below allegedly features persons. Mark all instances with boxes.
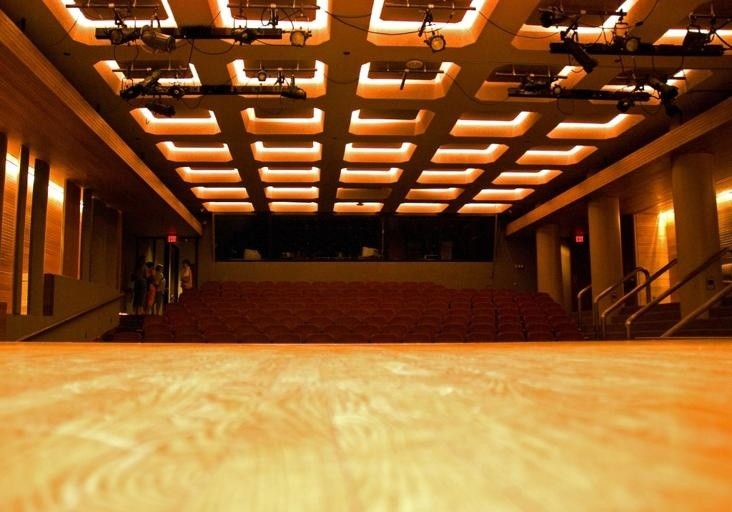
[133,255,167,315]
[180,258,193,293]
